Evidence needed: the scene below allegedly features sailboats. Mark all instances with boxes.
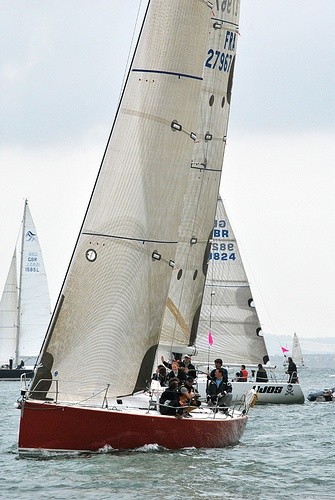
[1,197,52,381]
[15,1,248,454]
[284,332,308,371]
[190,193,307,406]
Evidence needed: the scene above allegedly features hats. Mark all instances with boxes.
[184,355,190,358]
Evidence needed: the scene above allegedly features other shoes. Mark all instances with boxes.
[187,414,191,417]
[223,410,229,414]
